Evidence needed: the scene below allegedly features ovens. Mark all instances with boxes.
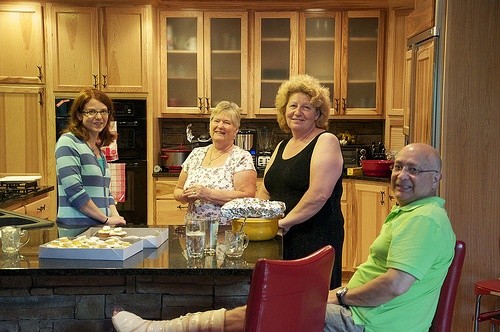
[107,160,148,227]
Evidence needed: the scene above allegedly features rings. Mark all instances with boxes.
[193,191,196,195]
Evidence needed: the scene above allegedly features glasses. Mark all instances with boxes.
[390,164,439,175]
[79,109,110,115]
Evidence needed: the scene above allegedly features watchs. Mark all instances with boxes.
[335,286,349,308]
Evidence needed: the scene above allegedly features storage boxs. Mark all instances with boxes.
[39,227,168,260]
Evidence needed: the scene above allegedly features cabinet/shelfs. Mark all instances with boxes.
[0,4,148,219]
[155,0,438,278]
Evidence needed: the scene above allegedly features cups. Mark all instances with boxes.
[186,219,209,260]
[1,225,31,253]
[224,230,249,258]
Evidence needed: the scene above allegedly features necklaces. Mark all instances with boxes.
[207,143,234,166]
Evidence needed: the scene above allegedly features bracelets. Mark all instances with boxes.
[100,216,108,224]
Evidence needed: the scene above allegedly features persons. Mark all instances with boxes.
[172,101,258,226]
[54,88,127,239]
[262,75,345,292]
[111,142,456,332]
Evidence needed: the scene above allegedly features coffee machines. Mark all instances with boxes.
[235,130,257,152]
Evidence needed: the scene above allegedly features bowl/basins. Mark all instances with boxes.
[231,216,283,241]
[360,159,394,176]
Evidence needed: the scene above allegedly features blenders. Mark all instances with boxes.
[256,127,273,175]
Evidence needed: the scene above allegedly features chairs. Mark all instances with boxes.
[244,245,335,332]
[429,240,466,332]
[474,279,500,332]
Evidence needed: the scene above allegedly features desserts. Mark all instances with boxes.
[45,225,132,249]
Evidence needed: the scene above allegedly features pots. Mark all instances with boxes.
[159,147,193,173]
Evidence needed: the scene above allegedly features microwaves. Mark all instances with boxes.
[55,100,147,162]
